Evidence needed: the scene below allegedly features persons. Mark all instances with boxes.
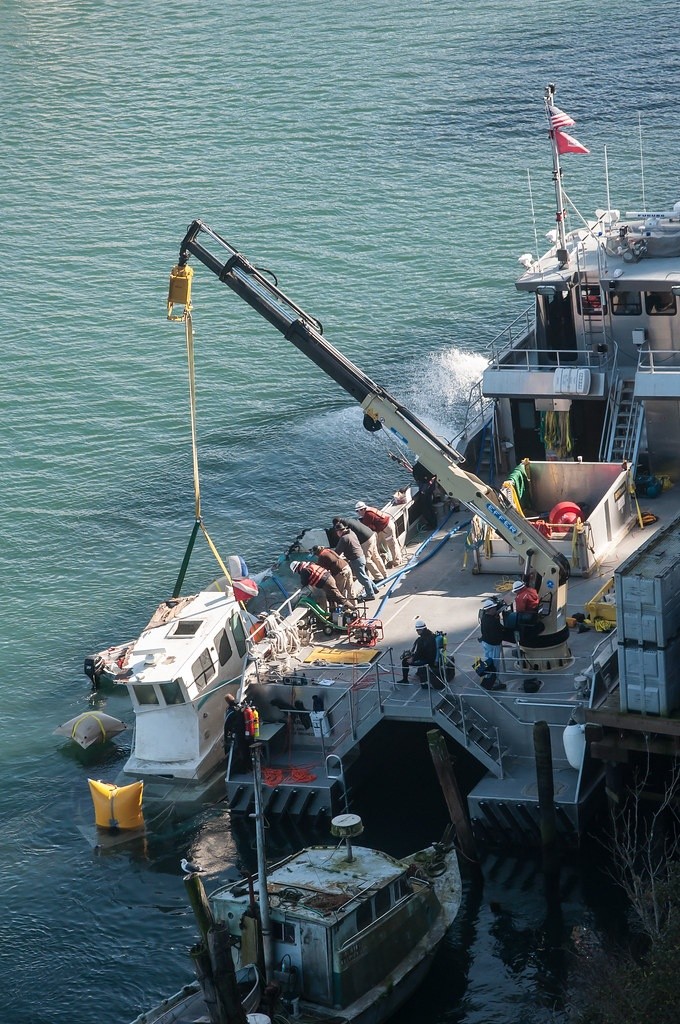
[480,600,507,691]
[290,501,402,621]
[507,581,543,643]
[413,460,438,532]
[397,620,437,687]
[223,694,253,775]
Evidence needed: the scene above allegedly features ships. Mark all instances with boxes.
[225,90,680,852]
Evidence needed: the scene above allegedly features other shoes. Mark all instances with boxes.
[372,579,382,583]
[361,595,376,600]
[387,561,399,566]
[396,678,409,683]
[494,684,506,691]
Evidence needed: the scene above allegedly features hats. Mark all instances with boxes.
[312,544,324,555]
[334,521,348,531]
[332,517,340,528]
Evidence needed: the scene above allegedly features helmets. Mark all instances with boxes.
[354,501,368,511]
[512,580,525,593]
[480,600,498,611]
[414,620,427,629]
[289,560,300,574]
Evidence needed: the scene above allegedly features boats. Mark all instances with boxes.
[87,556,279,826]
[130,741,463,1024]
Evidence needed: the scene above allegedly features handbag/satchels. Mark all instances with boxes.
[523,677,542,693]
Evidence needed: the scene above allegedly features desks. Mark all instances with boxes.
[254,722,287,766]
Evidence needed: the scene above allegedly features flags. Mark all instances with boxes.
[547,105,590,155]
